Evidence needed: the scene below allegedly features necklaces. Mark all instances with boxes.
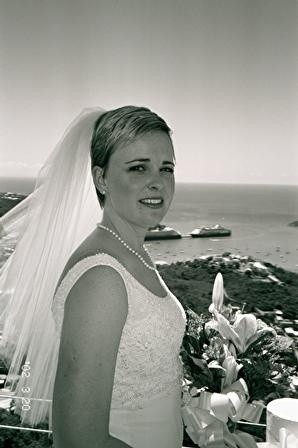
[98,223,156,270]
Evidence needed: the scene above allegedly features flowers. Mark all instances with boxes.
[178,272,298,448]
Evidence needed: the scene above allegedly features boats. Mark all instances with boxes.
[190,224,231,237]
[144,224,181,240]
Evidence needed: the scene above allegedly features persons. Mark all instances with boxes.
[0,105,187,448]
